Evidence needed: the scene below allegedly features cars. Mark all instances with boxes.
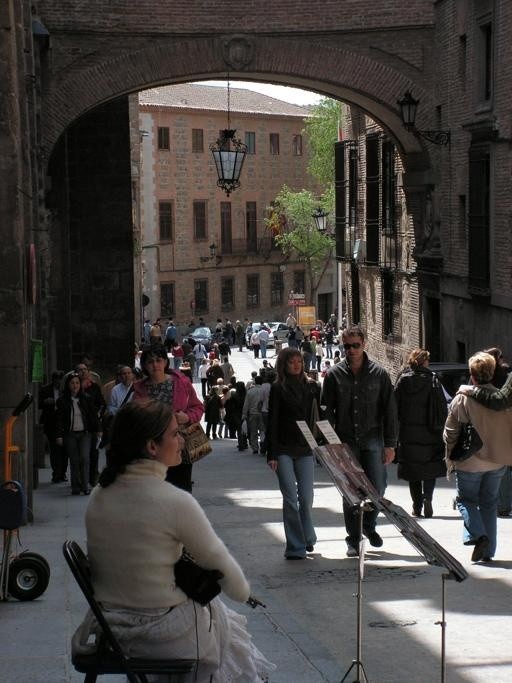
[181,325,227,352]
[308,325,326,343]
[242,320,268,344]
[245,321,290,347]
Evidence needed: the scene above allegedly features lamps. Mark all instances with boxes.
[312,205,336,243]
[209,66,248,195]
[395,88,451,151]
[199,240,218,262]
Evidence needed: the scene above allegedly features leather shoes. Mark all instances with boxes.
[471,535,489,561]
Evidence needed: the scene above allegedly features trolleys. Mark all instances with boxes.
[1,391,50,600]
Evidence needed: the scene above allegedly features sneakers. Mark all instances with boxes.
[363,523,383,547]
[346,536,359,557]
[423,498,432,518]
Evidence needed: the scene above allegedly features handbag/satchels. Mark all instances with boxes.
[179,421,213,464]
[449,423,483,462]
[309,398,323,443]
[174,546,224,606]
[429,378,447,434]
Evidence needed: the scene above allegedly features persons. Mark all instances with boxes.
[38,313,512,562]
[82,389,278,683]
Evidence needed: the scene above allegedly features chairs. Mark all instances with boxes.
[61,536,197,681]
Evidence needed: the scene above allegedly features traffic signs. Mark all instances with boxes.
[288,299,306,305]
[289,293,306,299]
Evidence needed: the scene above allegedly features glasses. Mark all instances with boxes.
[344,343,361,349]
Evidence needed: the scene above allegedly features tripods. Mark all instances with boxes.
[340,508,368,683]
